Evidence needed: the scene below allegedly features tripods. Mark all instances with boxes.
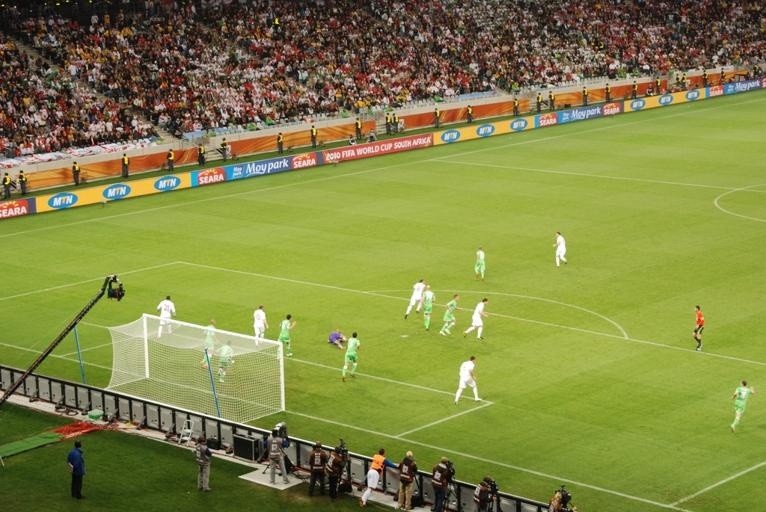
[262,448,301,479]
[443,478,458,512]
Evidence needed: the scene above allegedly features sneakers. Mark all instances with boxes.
[269,478,369,508]
[454,397,482,406]
[196,485,211,492]
[342,373,357,382]
[199,338,295,383]
[404,310,485,341]
